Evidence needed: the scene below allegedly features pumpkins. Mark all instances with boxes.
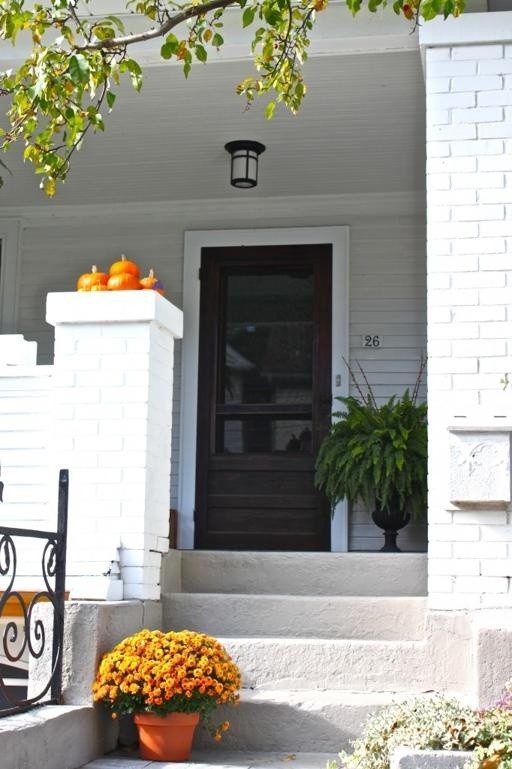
[76,254,165,296]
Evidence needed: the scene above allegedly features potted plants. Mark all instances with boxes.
[315,386,427,552]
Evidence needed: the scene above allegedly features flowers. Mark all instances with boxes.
[323,678,512,769]
[89,629,242,742]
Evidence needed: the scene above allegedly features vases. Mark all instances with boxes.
[133,713,200,761]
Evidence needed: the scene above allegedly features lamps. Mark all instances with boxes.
[224,139,266,188]
